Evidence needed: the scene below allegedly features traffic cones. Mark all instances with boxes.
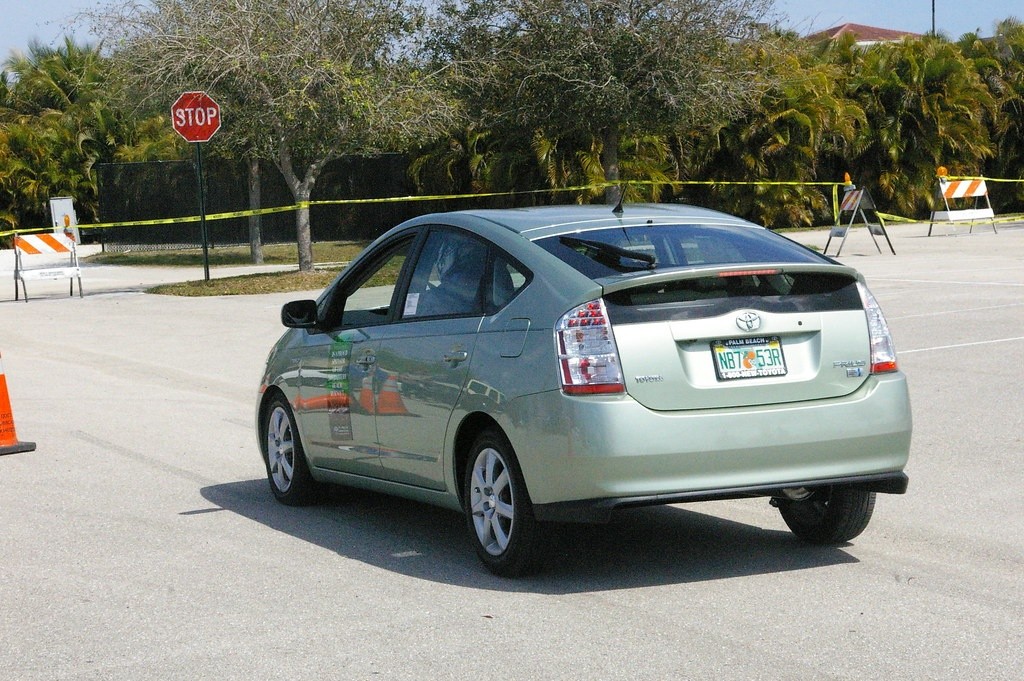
[0,374,39,458]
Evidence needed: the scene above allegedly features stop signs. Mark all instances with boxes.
[171,91,222,145]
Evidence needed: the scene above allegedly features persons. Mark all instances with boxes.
[416,234,514,315]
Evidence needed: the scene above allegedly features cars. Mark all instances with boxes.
[253,204,915,579]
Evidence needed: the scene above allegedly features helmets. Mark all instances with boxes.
[434,232,486,284]
